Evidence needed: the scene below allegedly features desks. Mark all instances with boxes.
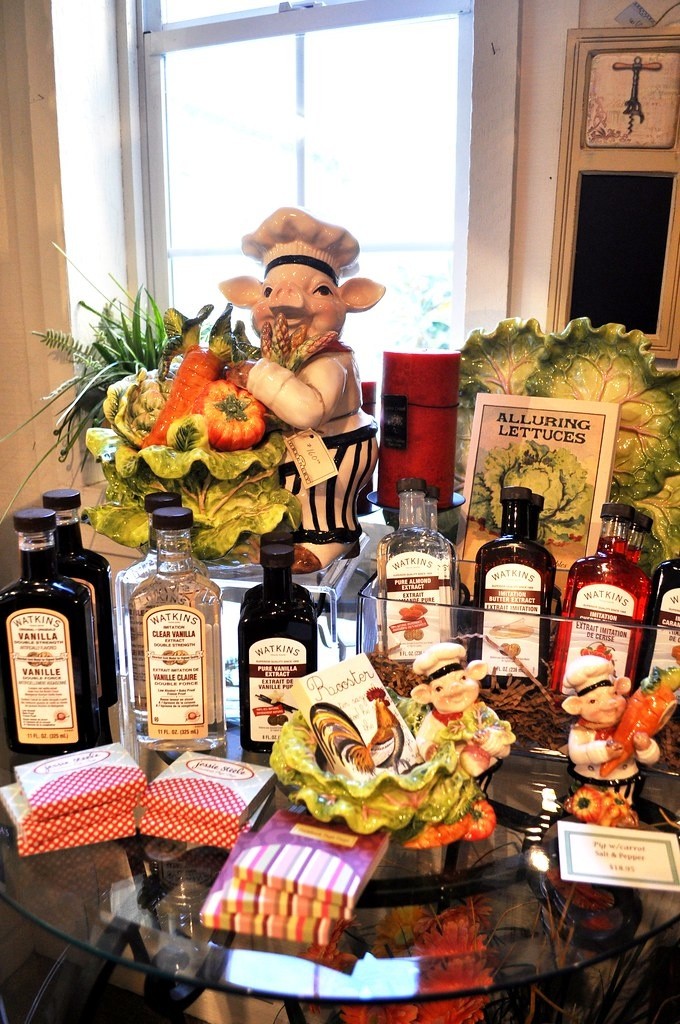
[0,675,680,1023]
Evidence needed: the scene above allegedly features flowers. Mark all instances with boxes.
[308,895,508,1024]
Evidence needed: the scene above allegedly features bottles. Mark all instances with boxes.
[0,488,320,754]
[371,476,680,727]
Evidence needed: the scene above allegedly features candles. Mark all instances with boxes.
[362,382,377,418]
[377,348,462,507]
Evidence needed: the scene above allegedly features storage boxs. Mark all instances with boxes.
[292,653,425,781]
[198,805,392,942]
[112,534,369,727]
[357,568,680,778]
[455,393,622,611]
[138,751,278,849]
[0,742,148,856]
